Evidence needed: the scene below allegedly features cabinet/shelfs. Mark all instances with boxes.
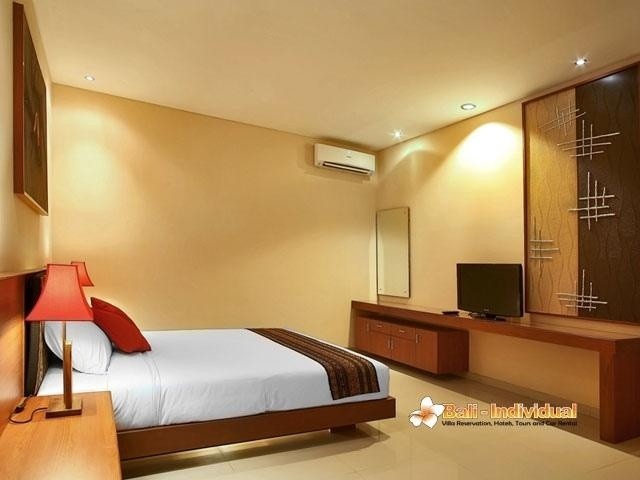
[351,313,471,379]
[351,299,640,444]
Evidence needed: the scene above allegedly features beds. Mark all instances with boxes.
[23,265,396,462]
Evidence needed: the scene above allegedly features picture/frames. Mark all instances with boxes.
[518,58,640,328]
[12,1,51,218]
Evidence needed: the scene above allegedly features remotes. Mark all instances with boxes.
[442,310,458,314]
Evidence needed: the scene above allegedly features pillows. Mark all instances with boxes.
[42,318,113,374]
[88,294,153,354]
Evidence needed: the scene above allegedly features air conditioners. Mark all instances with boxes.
[313,143,376,176]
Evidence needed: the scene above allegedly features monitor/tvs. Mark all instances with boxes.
[456,263,523,320]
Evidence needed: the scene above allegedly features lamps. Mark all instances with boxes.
[69,261,95,288]
[23,260,96,416]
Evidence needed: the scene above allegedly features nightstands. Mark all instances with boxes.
[0,387,126,480]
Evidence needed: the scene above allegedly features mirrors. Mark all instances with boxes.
[375,207,410,299]
